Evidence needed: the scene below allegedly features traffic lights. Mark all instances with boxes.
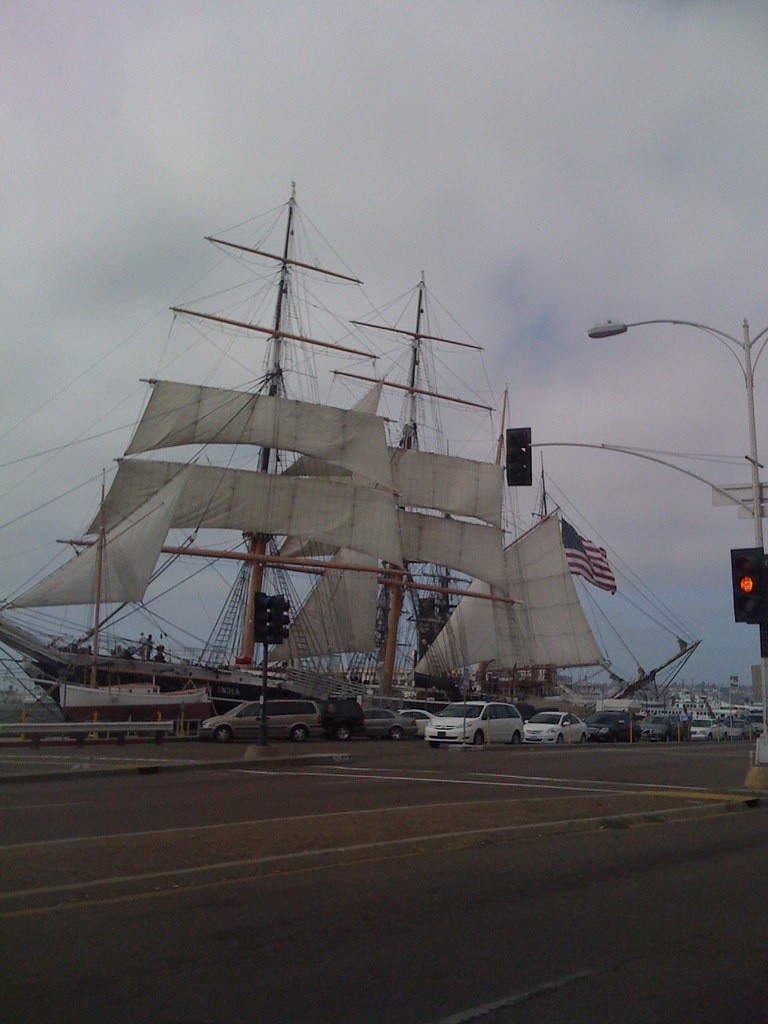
[271,594,291,645]
[254,591,273,642]
[729,546,768,624]
[505,426,533,487]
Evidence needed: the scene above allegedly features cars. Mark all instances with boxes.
[198,699,324,743]
[719,718,754,740]
[747,714,764,734]
[314,700,367,741]
[521,711,591,745]
[583,711,642,744]
[425,700,525,746]
[396,709,437,738]
[636,714,692,742]
[362,708,420,741]
[688,719,729,741]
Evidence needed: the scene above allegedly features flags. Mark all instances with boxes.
[562,518,618,595]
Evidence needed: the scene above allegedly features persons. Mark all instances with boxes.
[139,632,146,660]
[152,645,166,663]
[147,635,155,660]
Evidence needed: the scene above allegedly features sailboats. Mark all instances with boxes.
[0,173,705,713]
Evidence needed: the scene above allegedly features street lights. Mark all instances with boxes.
[586,317,768,737]
